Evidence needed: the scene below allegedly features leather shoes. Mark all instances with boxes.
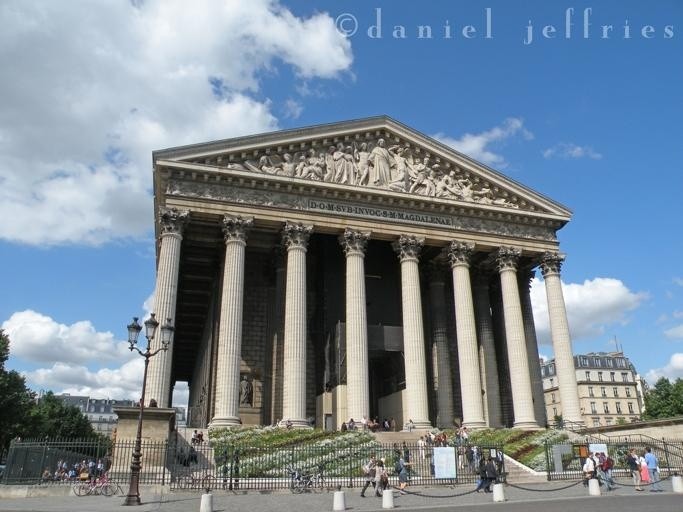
[636,489,644,491]
[477,490,493,492]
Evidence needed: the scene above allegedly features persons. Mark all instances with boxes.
[417,426,503,493]
[170,428,206,466]
[237,373,252,408]
[359,441,411,499]
[577,445,664,492]
[225,136,519,210]
[337,415,416,434]
[37,455,107,485]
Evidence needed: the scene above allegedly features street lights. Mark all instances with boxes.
[123,312,174,506]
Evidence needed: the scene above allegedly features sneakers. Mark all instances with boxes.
[400,489,409,495]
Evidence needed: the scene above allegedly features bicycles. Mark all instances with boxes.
[286,464,326,494]
[72,471,118,496]
[178,467,216,491]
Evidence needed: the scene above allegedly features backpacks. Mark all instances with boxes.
[395,464,401,472]
